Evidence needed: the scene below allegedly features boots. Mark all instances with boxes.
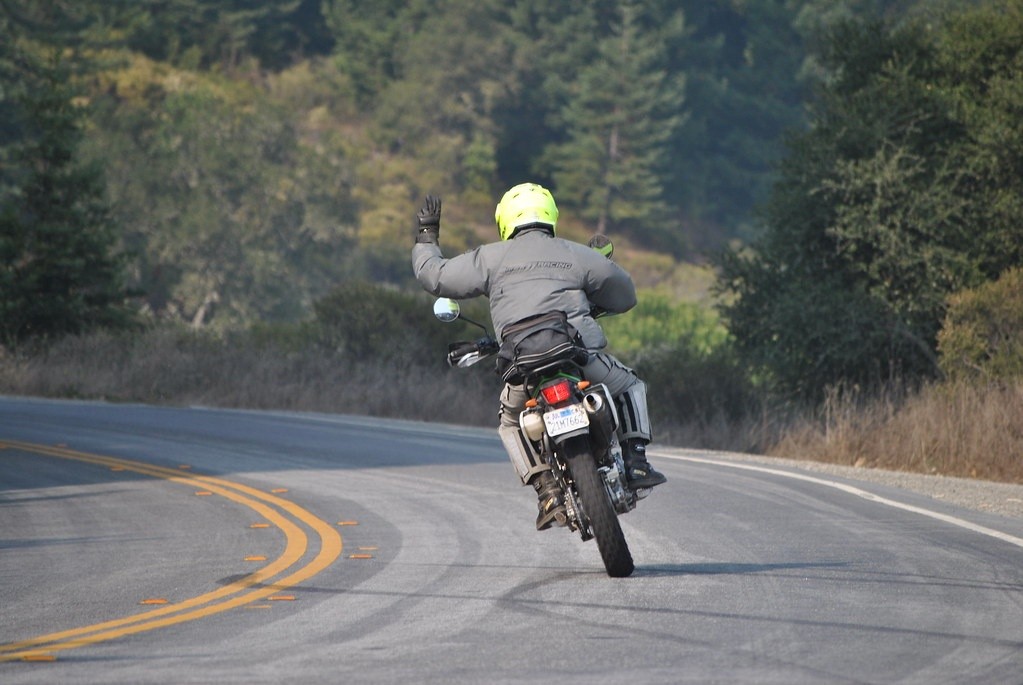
[531,470,567,530]
[620,439,667,488]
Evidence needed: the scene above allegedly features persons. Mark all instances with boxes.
[410,183,667,529]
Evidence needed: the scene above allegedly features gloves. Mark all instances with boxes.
[414,194,442,244]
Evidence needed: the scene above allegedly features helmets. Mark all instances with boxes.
[495,182,559,240]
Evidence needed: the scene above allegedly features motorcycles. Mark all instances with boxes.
[433,235,666,577]
